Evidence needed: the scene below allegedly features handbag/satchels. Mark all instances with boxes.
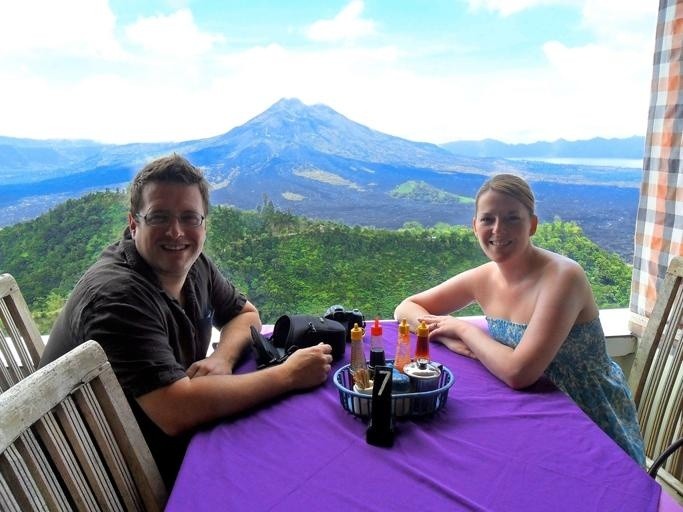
[244,311,348,372]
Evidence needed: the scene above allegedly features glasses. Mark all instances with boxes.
[133,208,207,227]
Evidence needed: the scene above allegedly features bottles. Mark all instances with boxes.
[393,317,412,370]
[350,322,368,372]
[369,319,386,367]
[414,321,431,362]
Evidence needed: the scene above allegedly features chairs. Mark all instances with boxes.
[0,340,167,512]
[626,256,683,507]
[0,271,45,394]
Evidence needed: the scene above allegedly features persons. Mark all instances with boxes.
[19,152,336,509]
[391,172,650,479]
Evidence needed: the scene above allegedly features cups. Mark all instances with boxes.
[403,359,444,412]
